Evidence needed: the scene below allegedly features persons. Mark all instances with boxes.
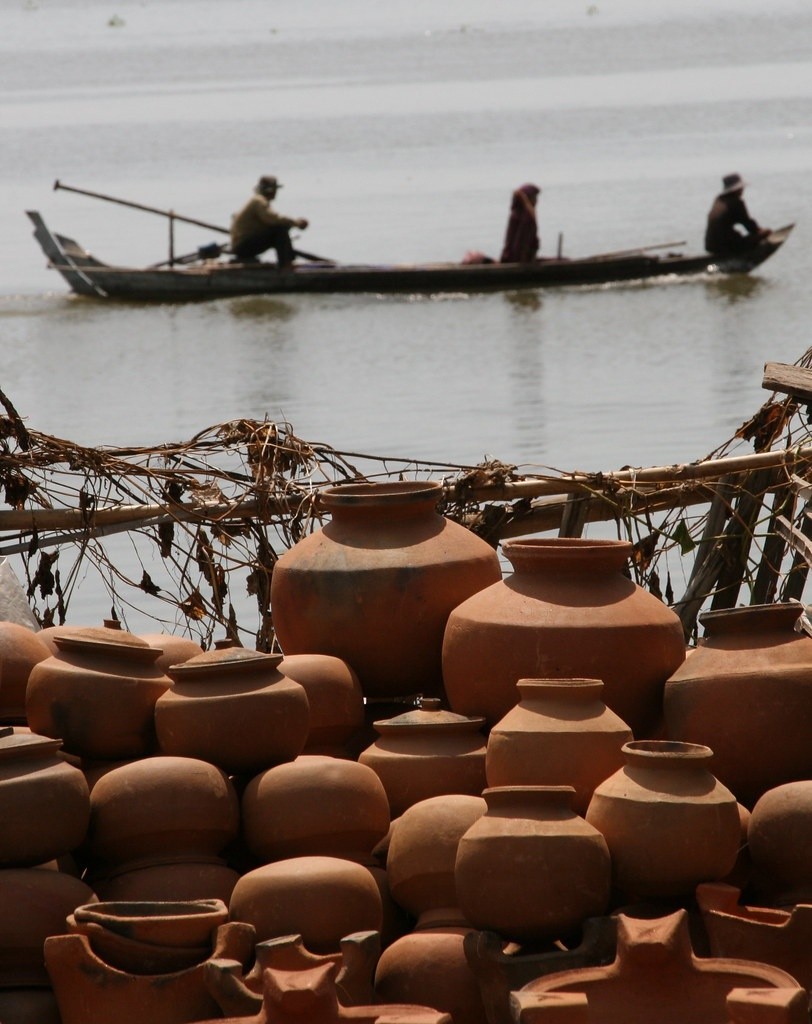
[501,184,540,263]
[231,176,308,266]
[704,172,763,250]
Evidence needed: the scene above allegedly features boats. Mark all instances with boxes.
[27,208,797,304]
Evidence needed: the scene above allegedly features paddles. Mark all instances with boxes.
[575,241,688,260]
[53,179,329,262]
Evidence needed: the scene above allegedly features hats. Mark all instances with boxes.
[716,173,749,199]
[261,177,282,190]
